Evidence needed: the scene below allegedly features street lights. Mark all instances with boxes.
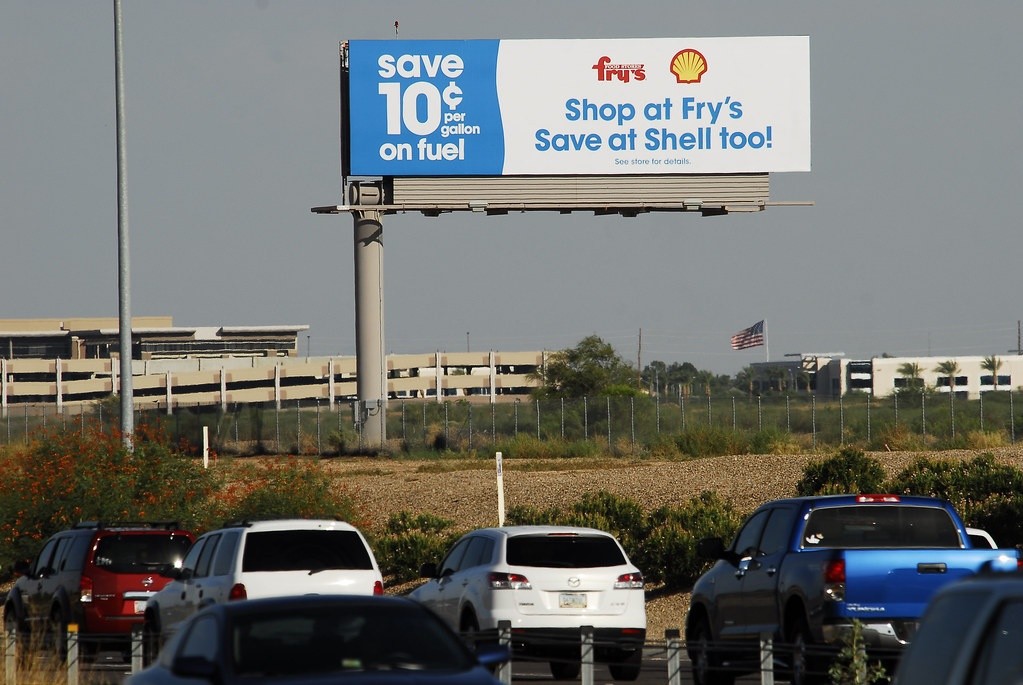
[467,332,471,352]
[306,335,310,357]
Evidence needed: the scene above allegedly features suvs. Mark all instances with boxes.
[408,525,646,681]
[4,522,198,662]
[142,518,387,666]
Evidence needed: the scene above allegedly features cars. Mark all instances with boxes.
[886,574,1023,685]
[123,592,509,685]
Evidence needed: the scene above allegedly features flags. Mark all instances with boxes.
[730,320,764,350]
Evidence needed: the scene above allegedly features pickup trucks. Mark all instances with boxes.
[682,495,1023,685]
